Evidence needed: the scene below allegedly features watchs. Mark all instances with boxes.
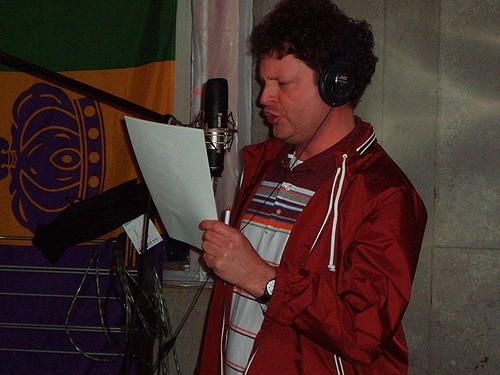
[255,276,277,304]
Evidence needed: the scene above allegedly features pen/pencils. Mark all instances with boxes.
[222,206,231,289]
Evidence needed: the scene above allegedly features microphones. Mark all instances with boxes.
[203,78,228,178]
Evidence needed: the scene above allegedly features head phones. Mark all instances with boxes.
[318,12,358,107]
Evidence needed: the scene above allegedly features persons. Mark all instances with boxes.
[193,0,428,375]
